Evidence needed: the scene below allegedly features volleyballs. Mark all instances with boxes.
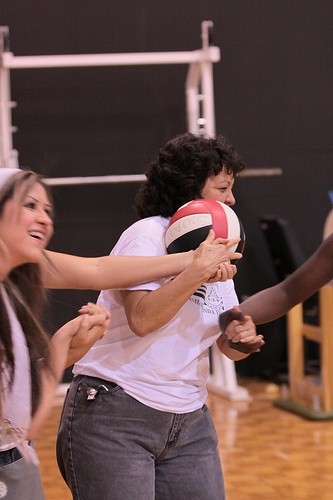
[164,199,245,262]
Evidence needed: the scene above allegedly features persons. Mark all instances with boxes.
[0,167,111,500]
[39,237,236,369]
[56,132,263,500]
[220,233,333,354]
